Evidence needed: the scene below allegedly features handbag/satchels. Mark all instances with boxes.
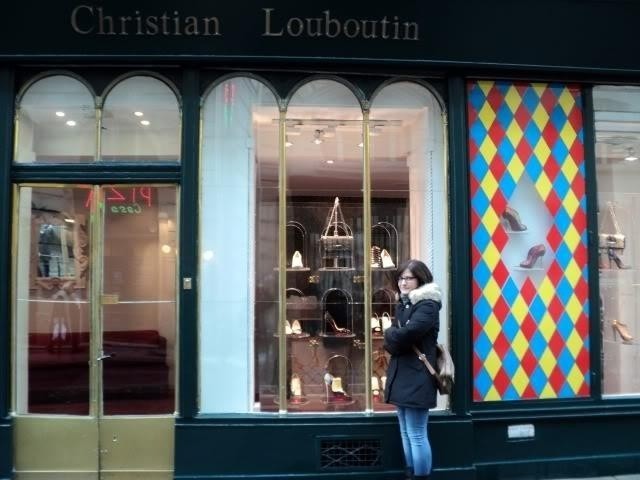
[597,200,626,249]
[435,344,456,396]
[319,196,355,260]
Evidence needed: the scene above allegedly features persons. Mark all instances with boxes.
[384,260,443,480]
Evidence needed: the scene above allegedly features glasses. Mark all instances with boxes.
[399,276,415,281]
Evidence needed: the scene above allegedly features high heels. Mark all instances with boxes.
[520,244,546,269]
[502,208,528,231]
[323,373,353,400]
[608,248,633,270]
[324,311,352,336]
[612,319,634,342]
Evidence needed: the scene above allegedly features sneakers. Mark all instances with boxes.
[289,373,302,395]
[292,320,302,334]
[381,249,395,268]
[372,376,386,396]
[286,319,292,335]
[291,250,303,269]
[371,246,382,269]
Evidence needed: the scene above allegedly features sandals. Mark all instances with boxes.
[382,312,392,336]
[371,312,382,336]
